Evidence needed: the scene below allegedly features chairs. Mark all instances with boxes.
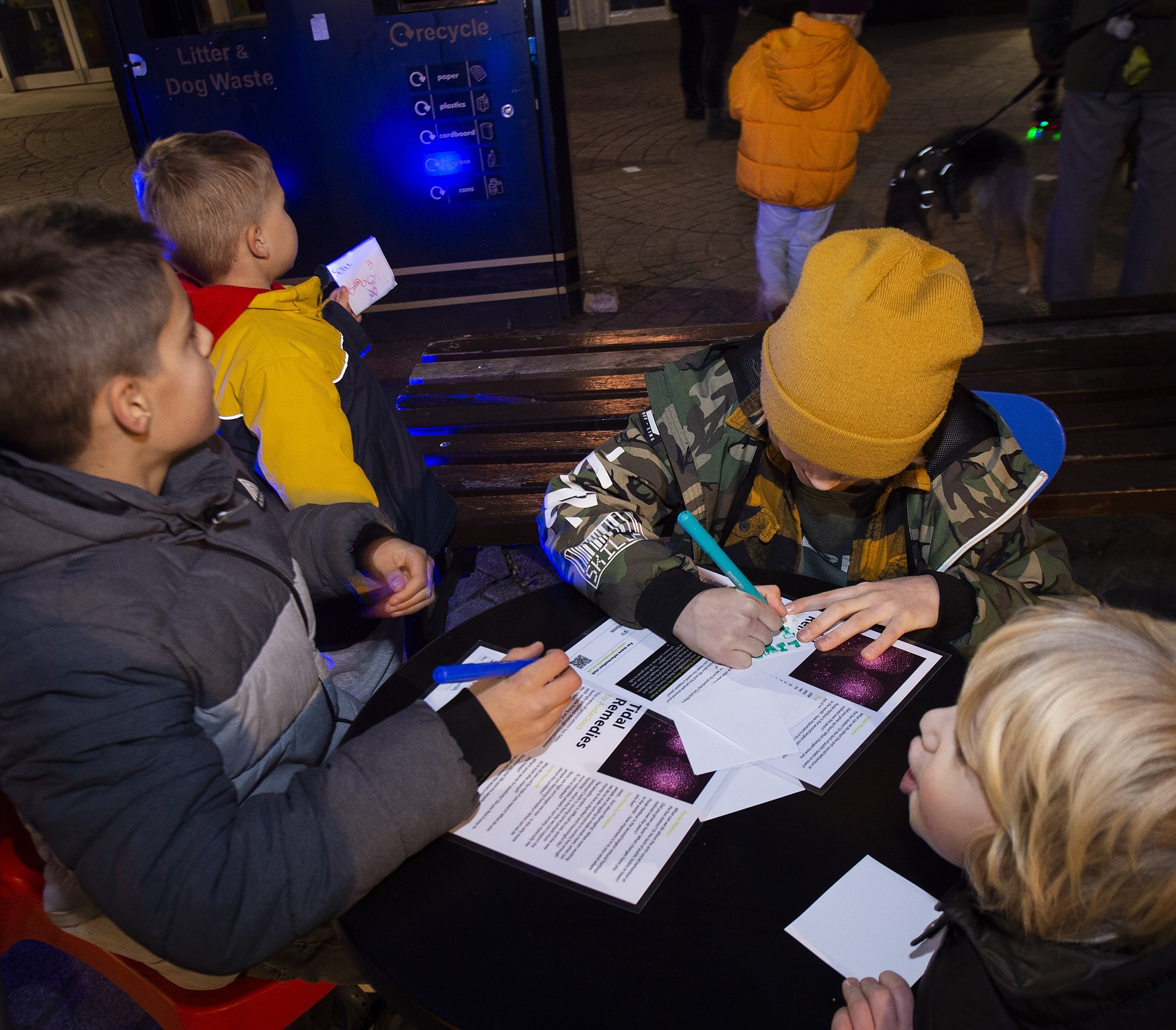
[0,787,340,1030]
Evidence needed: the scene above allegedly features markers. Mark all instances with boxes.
[677,511,787,632]
[432,658,541,683]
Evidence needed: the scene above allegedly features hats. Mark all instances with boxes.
[761,228,983,480]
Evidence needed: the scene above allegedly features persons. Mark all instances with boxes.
[727,0,890,322]
[0,134,583,977]
[133,133,461,640]
[1017,0,1176,330]
[670,0,742,140]
[535,228,1071,668]
[828,597,1176,1030]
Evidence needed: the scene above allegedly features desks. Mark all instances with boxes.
[328,569,1105,1030]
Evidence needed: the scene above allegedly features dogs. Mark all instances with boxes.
[884,126,1044,297]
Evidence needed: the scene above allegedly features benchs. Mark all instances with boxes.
[350,309,1175,559]
